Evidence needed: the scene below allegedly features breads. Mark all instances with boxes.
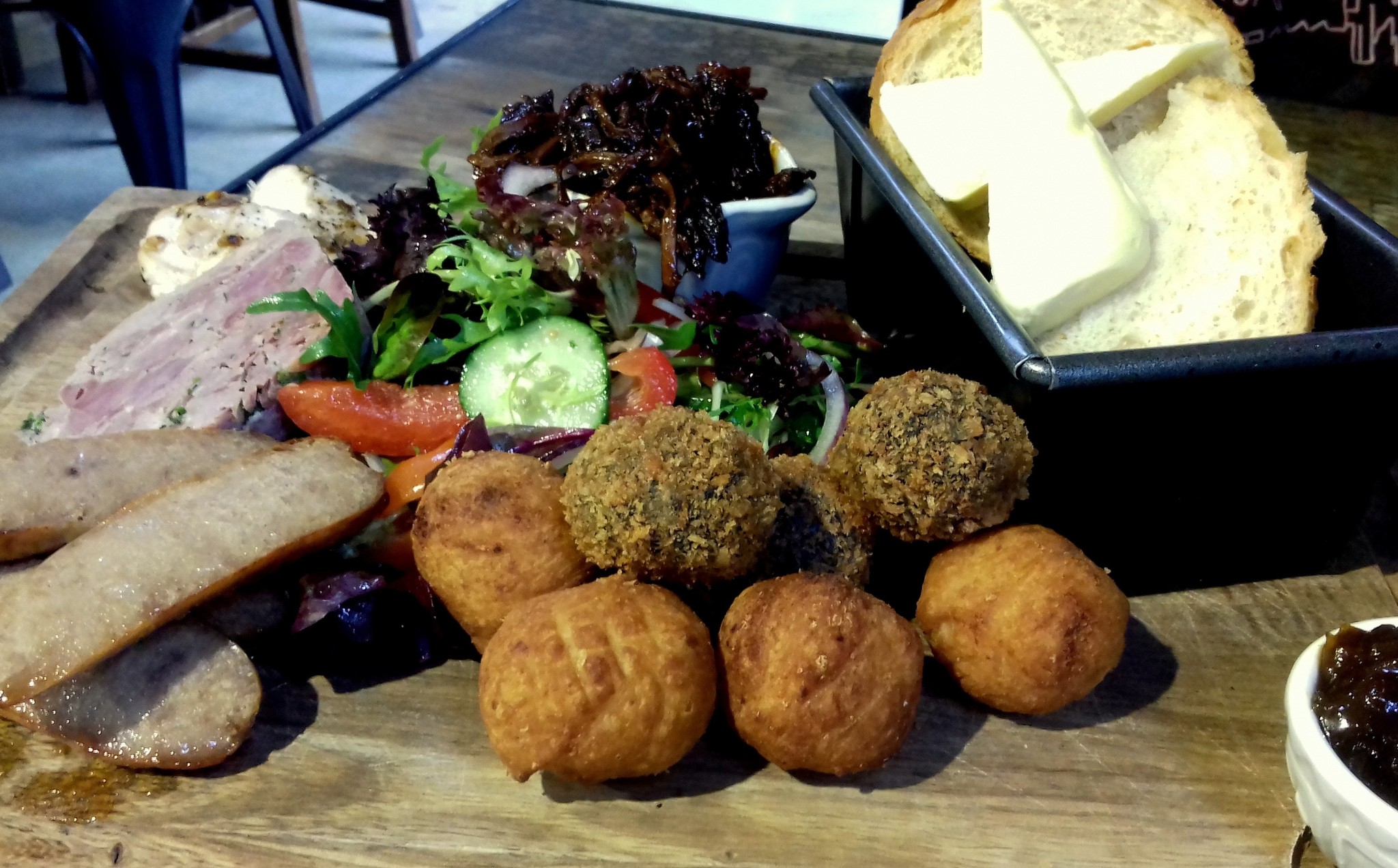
[862,0,1328,360]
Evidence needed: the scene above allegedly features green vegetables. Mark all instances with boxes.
[248,111,837,451]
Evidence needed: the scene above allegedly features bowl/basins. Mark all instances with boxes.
[806,63,1397,525]
[495,128,818,309]
[1281,609,1397,868]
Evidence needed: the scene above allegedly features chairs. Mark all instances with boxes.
[1,1,316,201]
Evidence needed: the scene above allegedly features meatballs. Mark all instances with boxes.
[406,368,1131,787]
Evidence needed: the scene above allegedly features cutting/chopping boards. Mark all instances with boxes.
[2,176,1394,862]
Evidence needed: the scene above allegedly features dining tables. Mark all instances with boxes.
[0,2,1398,868]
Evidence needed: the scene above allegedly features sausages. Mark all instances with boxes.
[0,424,388,772]
